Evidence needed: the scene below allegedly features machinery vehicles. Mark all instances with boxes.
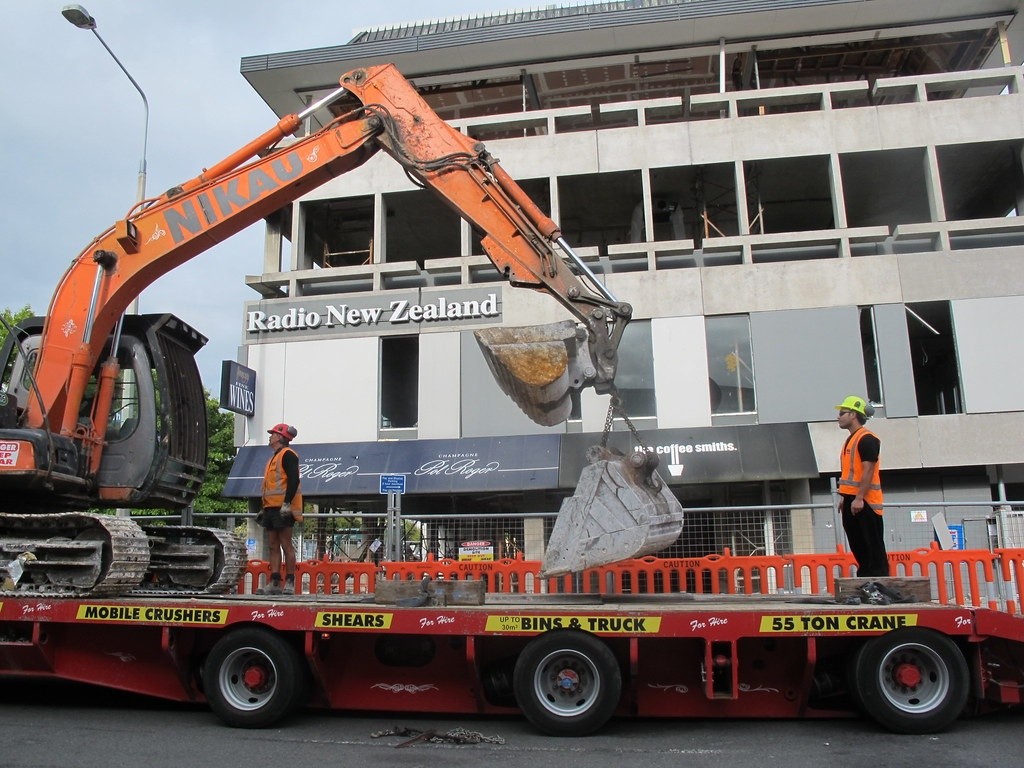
[0,53,633,596]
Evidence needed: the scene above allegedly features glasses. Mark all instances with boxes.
[839,410,852,416]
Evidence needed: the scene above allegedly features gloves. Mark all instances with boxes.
[280,502,291,520]
[254,510,265,528]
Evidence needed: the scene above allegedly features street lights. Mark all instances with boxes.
[60,4,151,315]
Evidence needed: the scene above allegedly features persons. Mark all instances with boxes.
[255,424,303,595]
[834,396,890,577]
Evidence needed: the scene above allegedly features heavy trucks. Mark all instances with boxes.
[0,598,1023,736]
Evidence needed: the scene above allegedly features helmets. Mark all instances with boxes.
[267,423,295,441]
[834,395,868,420]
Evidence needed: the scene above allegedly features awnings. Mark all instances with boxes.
[220,421,819,497]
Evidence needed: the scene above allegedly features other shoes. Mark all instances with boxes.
[283,578,295,595]
[255,580,282,595]
